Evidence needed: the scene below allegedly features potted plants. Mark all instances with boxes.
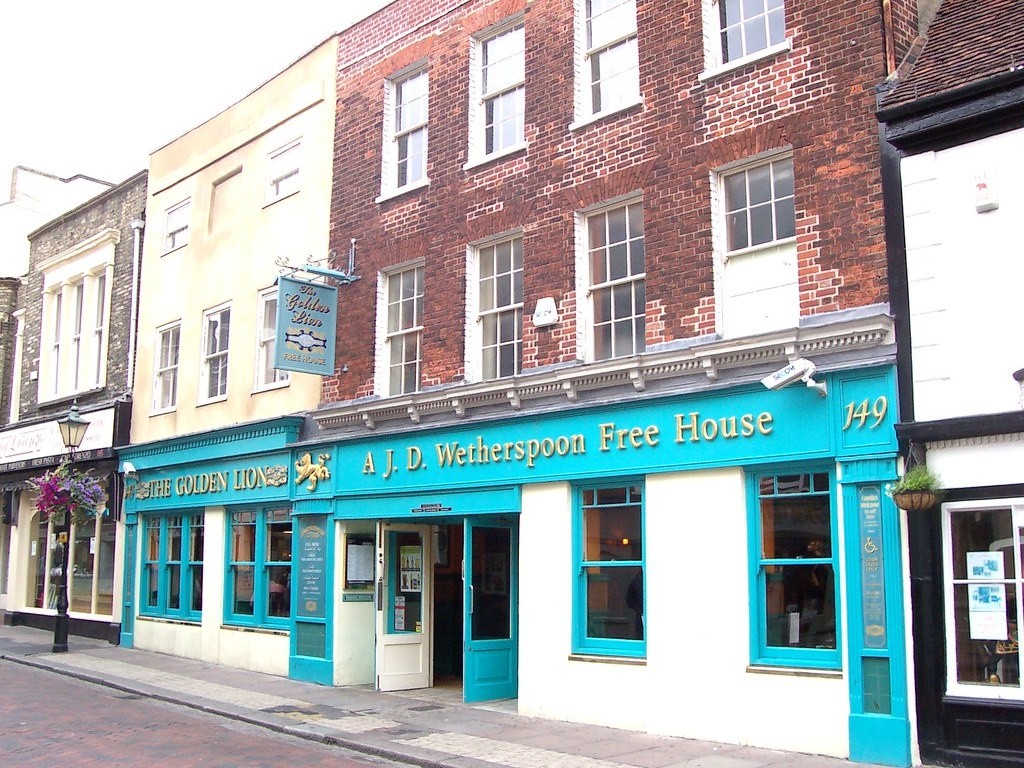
[890,463,945,510]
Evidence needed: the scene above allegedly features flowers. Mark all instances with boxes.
[23,458,112,528]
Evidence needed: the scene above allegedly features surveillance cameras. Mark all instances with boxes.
[759,358,815,391]
[123,462,137,476]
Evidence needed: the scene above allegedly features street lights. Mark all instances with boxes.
[51,398,91,653]
